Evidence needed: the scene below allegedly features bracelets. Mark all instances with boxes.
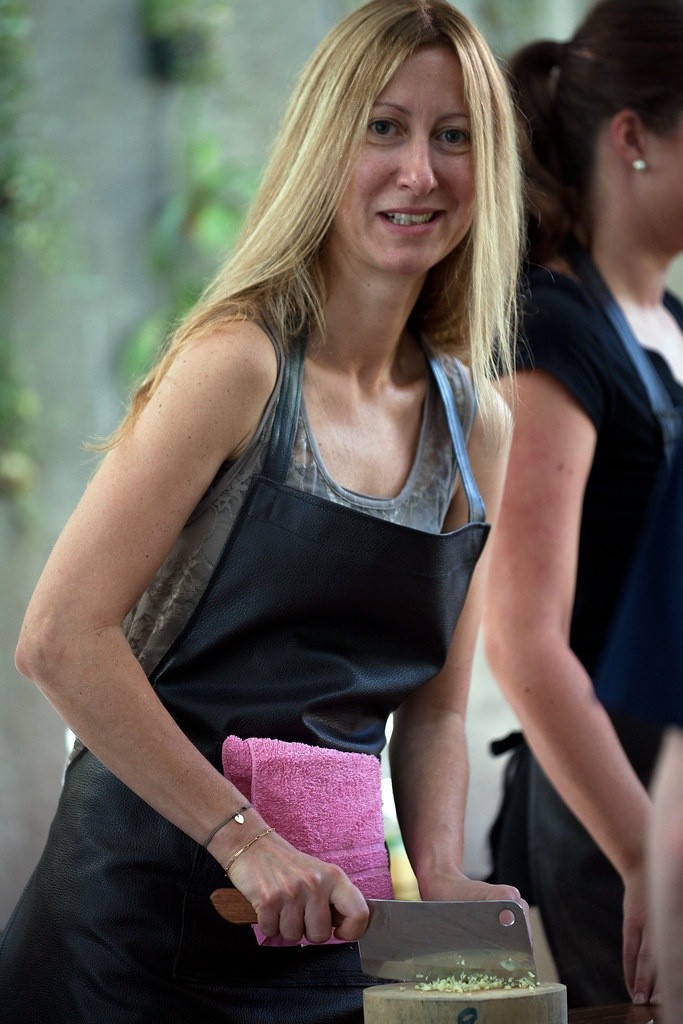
[204,804,254,850]
[225,826,277,875]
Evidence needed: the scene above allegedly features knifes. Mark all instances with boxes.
[211,888,540,990]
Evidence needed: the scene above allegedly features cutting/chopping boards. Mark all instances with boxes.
[361,982,567,1023]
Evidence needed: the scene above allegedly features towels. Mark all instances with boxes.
[221,734,394,946]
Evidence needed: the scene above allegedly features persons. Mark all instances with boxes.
[480,0,683,1024]
[0,0,533,1024]
